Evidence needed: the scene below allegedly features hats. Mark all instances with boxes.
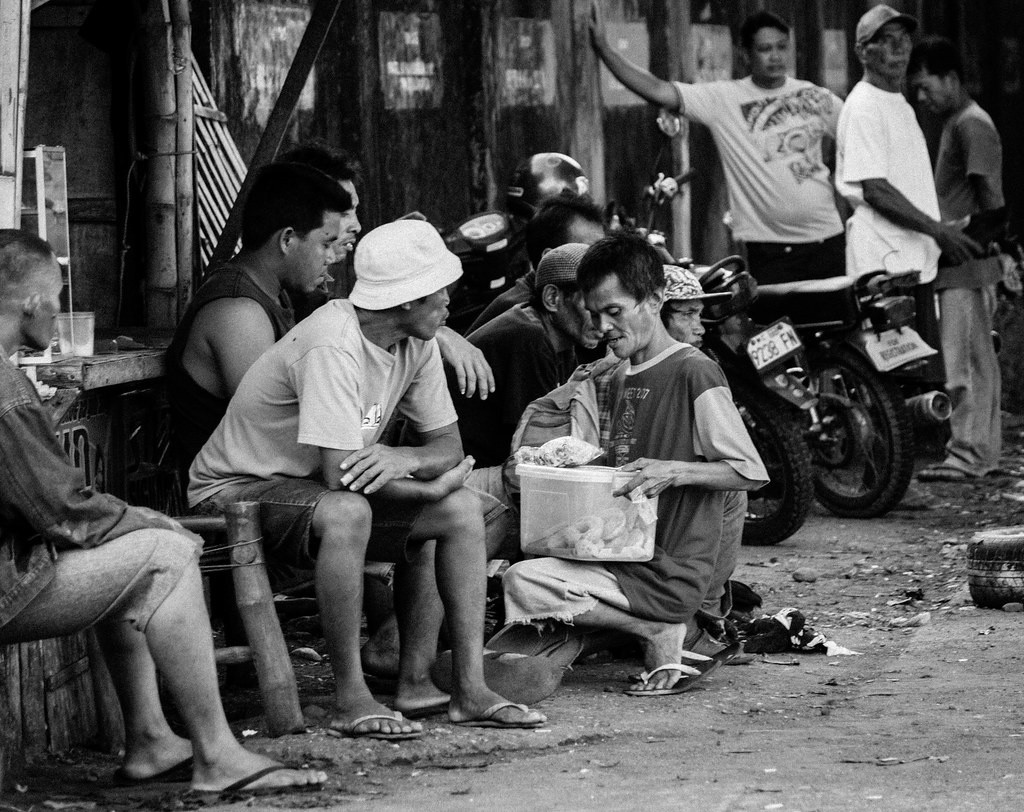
[349,218,463,309]
[533,241,589,290]
[660,263,732,312]
[856,4,919,45]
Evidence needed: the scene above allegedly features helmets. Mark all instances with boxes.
[504,152,592,229]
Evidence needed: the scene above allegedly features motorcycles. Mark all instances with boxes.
[436,108,953,547]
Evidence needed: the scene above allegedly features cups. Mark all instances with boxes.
[59,312,95,358]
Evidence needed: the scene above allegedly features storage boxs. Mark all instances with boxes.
[515,461,657,563]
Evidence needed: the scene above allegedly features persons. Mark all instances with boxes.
[0,227,328,796]
[258,140,509,680]
[394,189,771,694]
[585,0,1024,482]
[186,219,548,740]
[144,162,453,715]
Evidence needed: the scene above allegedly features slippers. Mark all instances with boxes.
[449,704,545,727]
[687,627,757,665]
[625,642,743,696]
[113,751,193,784]
[328,711,426,739]
[194,764,323,796]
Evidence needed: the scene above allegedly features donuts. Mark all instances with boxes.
[546,505,652,558]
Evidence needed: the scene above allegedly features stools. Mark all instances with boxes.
[163,500,403,734]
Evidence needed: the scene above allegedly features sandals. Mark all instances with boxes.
[918,462,970,481]
